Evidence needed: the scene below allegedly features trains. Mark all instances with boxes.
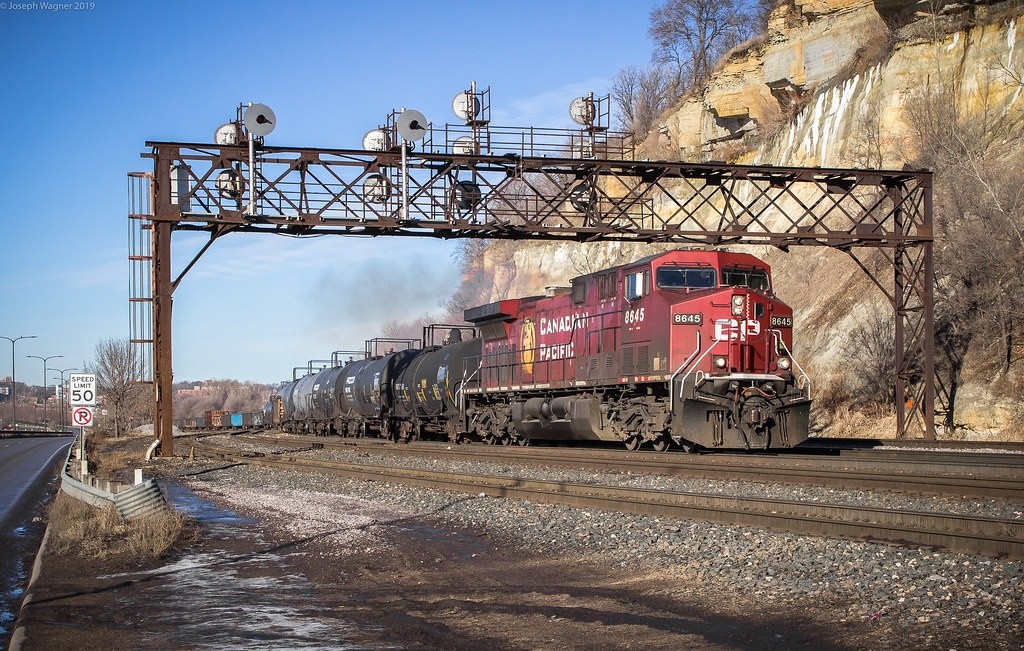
[172,245,815,456]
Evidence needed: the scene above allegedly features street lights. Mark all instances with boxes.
[25,355,65,432]
[46,367,77,431]
[0,335,38,430]
